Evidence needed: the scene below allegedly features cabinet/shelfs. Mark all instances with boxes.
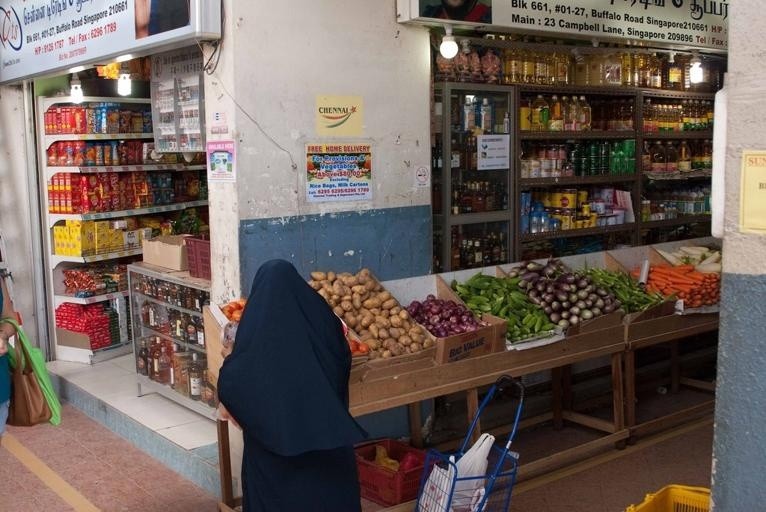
[39,96,207,365]
[431,33,726,271]
[127,262,218,421]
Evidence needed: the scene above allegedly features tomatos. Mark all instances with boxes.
[222,299,369,355]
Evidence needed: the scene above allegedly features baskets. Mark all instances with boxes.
[186,234,210,280]
[625,484,711,512]
[354,439,442,508]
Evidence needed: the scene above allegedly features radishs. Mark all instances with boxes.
[656,246,722,273]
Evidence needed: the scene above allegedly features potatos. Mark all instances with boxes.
[307,269,433,359]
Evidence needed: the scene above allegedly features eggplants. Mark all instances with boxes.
[507,262,623,329]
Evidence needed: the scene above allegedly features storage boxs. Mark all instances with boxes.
[311,246,678,382]
[143,235,192,270]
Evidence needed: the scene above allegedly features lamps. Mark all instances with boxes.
[117,62,132,96]
[70,73,84,103]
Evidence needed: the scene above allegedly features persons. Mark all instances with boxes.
[423,0,492,24]
[213,260,364,511]
[0,275,18,435]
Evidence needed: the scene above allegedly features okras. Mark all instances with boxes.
[576,266,664,312]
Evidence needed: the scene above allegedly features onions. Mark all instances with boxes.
[406,294,485,337]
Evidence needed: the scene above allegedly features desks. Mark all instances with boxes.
[625,313,718,444]
[208,306,624,512]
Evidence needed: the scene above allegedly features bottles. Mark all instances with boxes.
[135,274,209,406]
[433,49,714,268]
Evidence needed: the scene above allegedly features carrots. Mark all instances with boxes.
[631,265,721,307]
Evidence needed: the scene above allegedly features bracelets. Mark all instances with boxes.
[0,329,10,341]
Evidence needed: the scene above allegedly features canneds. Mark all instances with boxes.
[529,188,577,234]
[520,142,609,180]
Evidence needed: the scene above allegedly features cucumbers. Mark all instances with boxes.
[451,272,554,342]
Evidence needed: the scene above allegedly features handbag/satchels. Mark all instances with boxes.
[5,322,52,428]
[0,318,62,426]
